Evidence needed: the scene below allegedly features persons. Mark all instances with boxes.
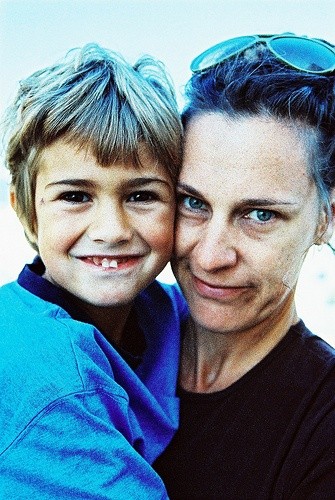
[154,33,334,500]
[0,42,190,500]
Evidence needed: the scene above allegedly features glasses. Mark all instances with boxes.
[191,34,335,75]
